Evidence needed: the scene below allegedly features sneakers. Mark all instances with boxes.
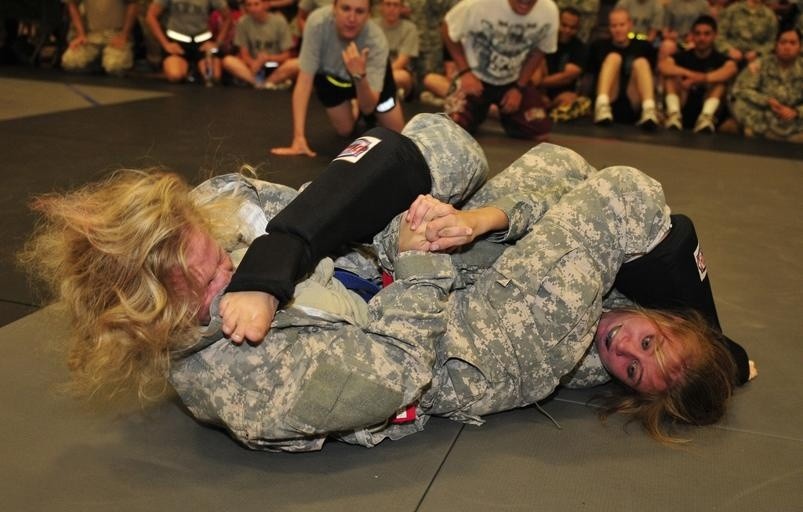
[640,106,716,131]
[595,103,613,124]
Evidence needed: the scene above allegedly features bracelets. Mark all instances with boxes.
[459,67,470,77]
[514,82,527,94]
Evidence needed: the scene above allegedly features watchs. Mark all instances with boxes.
[352,72,366,82]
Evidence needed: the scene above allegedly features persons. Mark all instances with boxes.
[216,108,765,448]
[14,142,765,456]
[271,1,405,159]
[443,0,560,140]
[60,0,420,100]
[421,2,802,141]
